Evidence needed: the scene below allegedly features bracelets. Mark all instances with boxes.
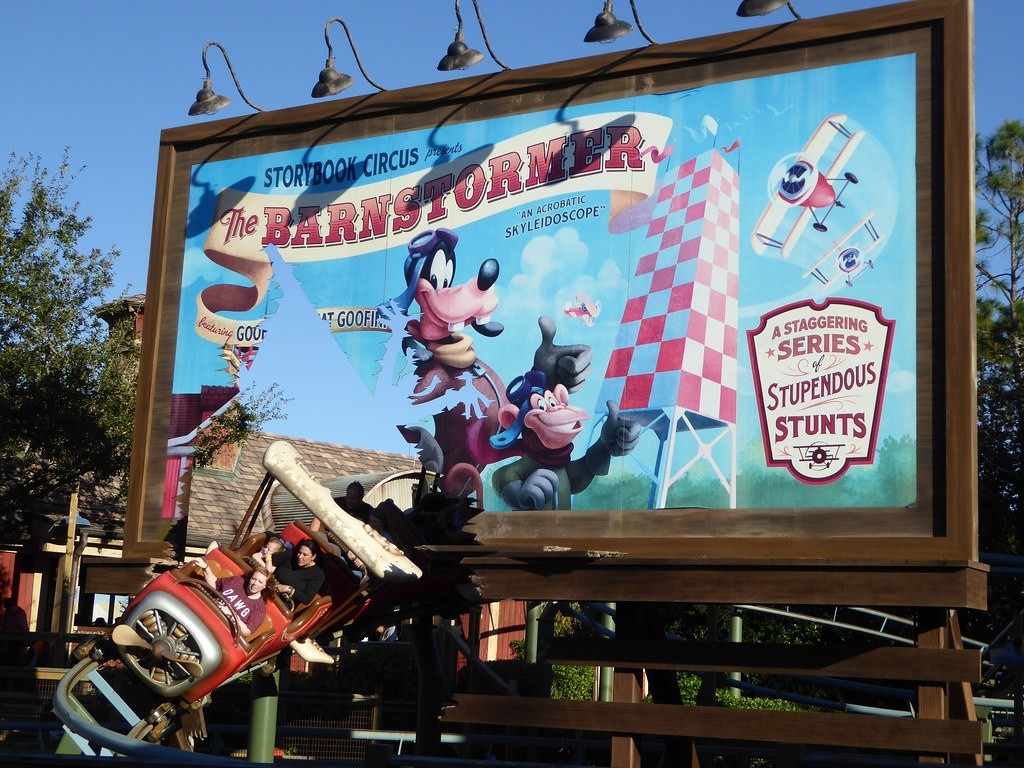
[287,586,293,594]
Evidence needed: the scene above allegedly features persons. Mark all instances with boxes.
[252,536,287,576]
[266,537,326,615]
[210,567,278,643]
[331,482,412,585]
[0,598,30,691]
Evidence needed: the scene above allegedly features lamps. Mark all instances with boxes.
[310,17,384,99]
[188,42,263,117]
[584,0,658,45]
[736,0,800,19]
[437,0,510,71]
